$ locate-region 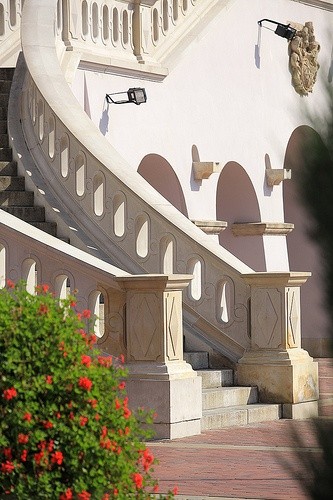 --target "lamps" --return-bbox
[106,87,147,105]
[258,19,297,42]
